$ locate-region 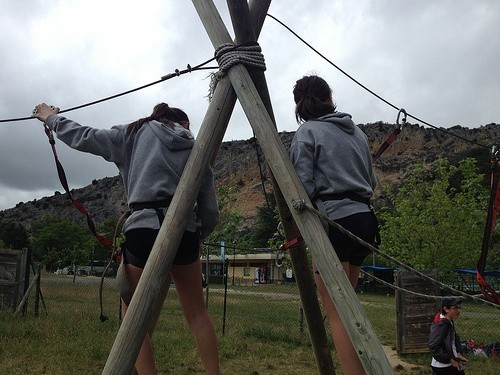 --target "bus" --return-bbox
[60,259,116,276]
